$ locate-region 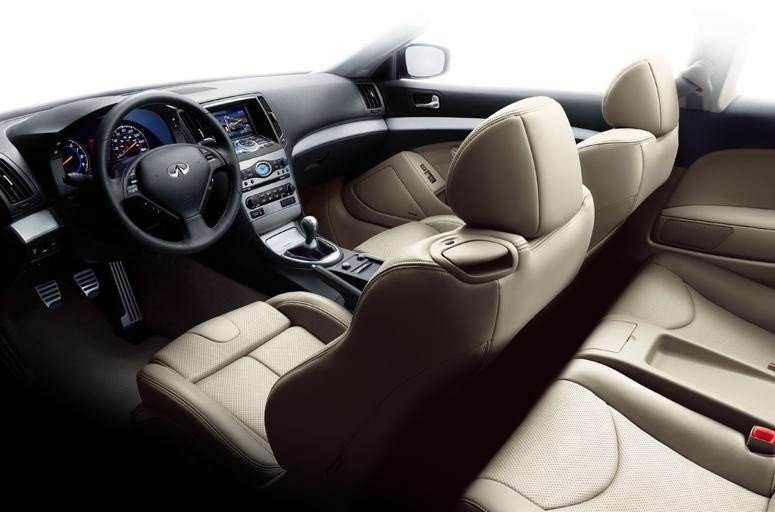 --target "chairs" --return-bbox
[132,92,595,509]
[353,50,683,265]
[462,252,773,511]
